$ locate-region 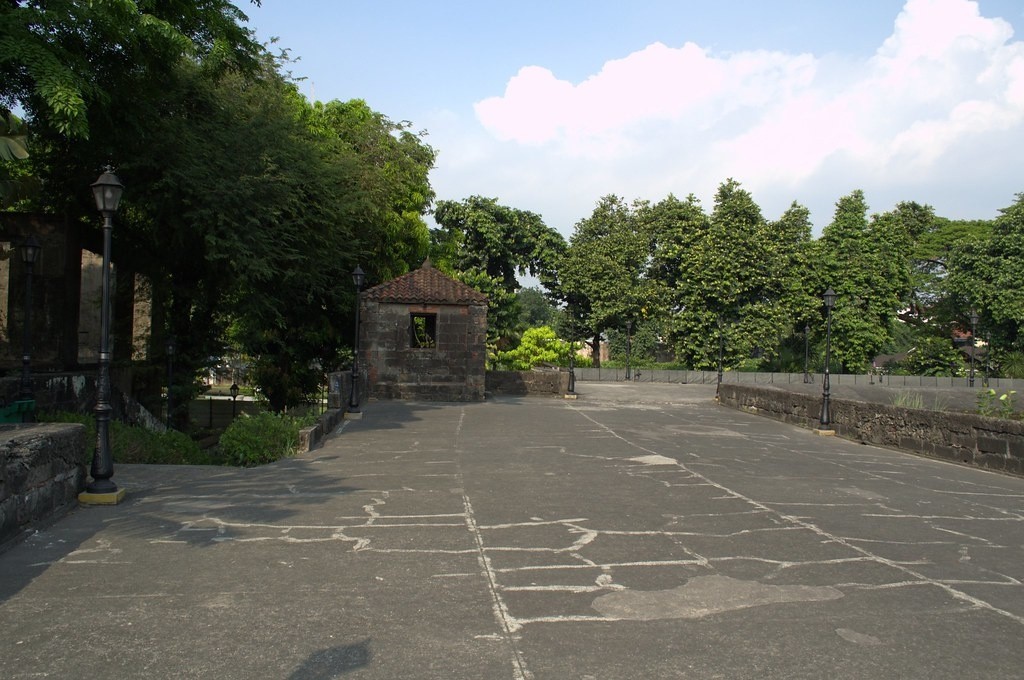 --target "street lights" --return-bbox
[812,284,837,436]
[343,263,365,420]
[76,163,127,506]
[625,316,632,382]
[984,330,991,387]
[565,307,578,398]
[714,295,726,402]
[803,323,810,383]
[969,311,980,388]
[230,381,240,420]
